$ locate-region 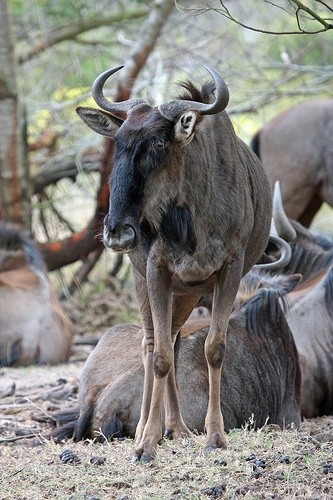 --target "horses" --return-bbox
[76,62,274,464]
[2,219,71,369]
[50,98,332,441]
